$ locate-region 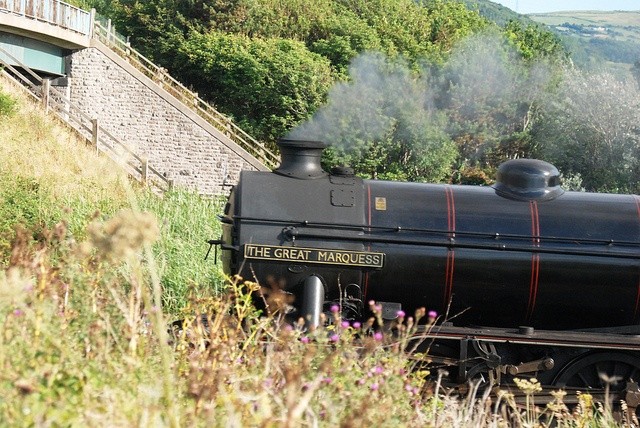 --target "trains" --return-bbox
[207,132,640,427]
